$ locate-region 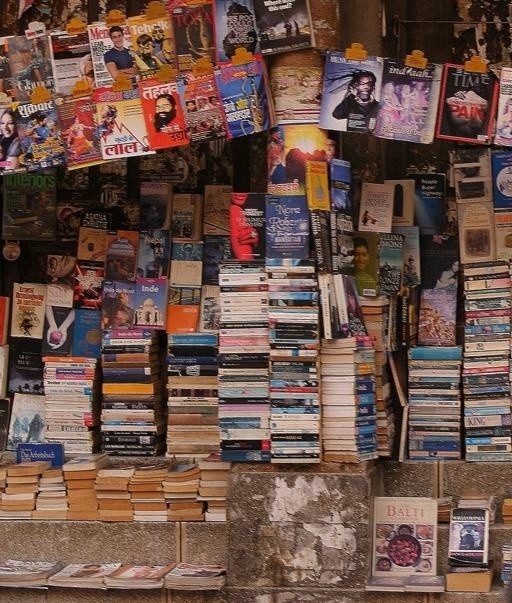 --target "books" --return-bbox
[366,496,512,592]
[306,138,511,463]
[1,133,323,464]
[0,0,316,175]
[0,559,227,589]
[318,51,511,147]
[0,443,232,523]
